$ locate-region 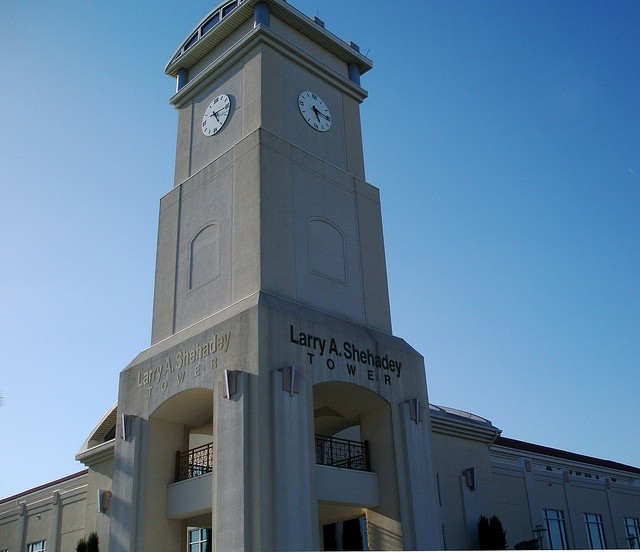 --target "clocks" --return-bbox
[201,94,231,136]
[298,91,332,131]
